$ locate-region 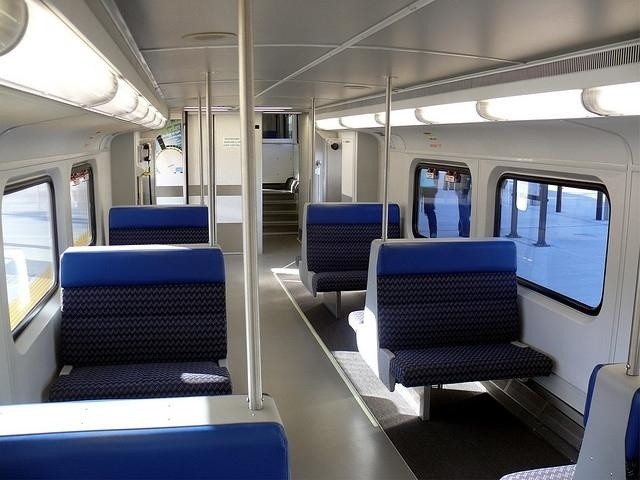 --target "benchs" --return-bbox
[40,206,230,404]
[499,359,640,480]
[347,236,556,420]
[294,202,402,319]
